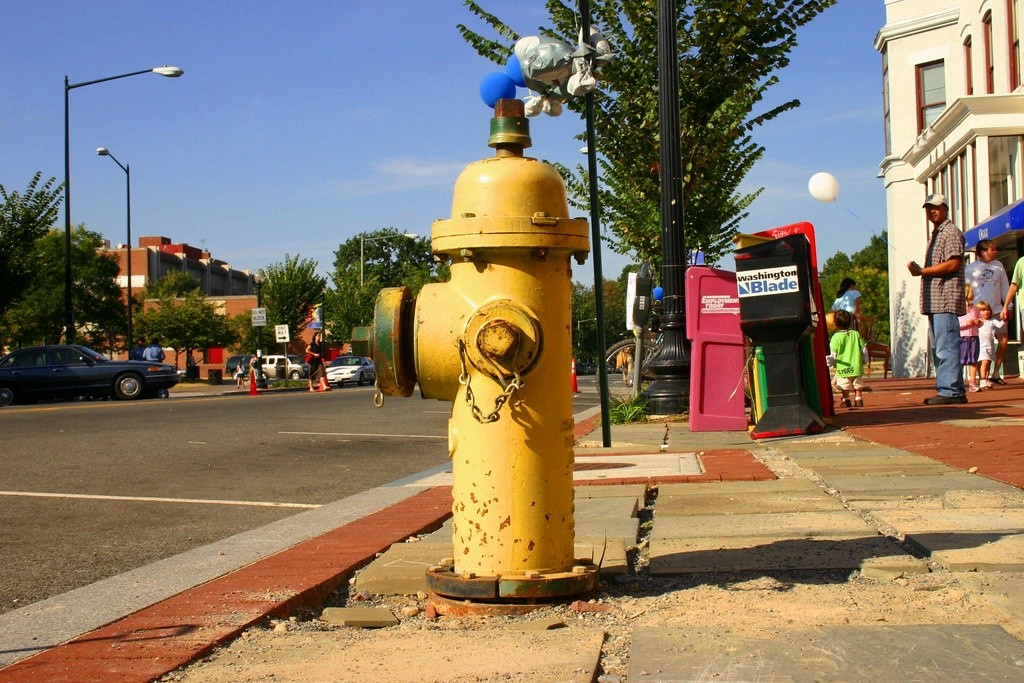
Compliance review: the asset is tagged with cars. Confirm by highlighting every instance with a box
[325,356,376,388]
[0,344,182,408]
[171,366,187,382]
[225,354,255,377]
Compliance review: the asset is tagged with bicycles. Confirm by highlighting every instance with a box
[596,332,665,411]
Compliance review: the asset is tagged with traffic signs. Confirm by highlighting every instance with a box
[275,324,290,343]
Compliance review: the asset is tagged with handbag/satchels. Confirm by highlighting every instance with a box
[305,353,312,361]
[234,373,238,380]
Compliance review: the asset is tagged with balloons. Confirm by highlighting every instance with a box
[651,287,665,304]
[479,53,537,112]
[808,171,841,202]
[824,312,840,332]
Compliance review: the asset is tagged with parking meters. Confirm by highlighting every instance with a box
[632,277,652,399]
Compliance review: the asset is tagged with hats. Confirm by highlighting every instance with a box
[922,194,949,210]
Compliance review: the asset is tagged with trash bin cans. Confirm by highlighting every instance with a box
[275,362,286,380]
[207,369,223,386]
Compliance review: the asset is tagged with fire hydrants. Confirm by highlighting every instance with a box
[351,97,601,618]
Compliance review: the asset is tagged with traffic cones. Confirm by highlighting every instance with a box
[571,357,582,394]
[247,370,261,396]
[317,358,331,391]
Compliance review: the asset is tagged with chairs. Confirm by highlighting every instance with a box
[52,351,65,365]
[860,315,890,379]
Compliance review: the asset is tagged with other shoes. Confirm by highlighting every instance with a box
[924,395,967,405]
[969,383,982,392]
[840,400,851,407]
[324,386,330,391]
[855,400,863,406]
[989,378,1007,385]
[980,380,993,388]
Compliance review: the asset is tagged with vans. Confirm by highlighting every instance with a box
[261,354,311,380]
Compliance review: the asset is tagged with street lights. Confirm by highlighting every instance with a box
[254,271,269,389]
[96,146,132,361]
[64,64,185,345]
[360,233,420,287]
[578,318,597,347]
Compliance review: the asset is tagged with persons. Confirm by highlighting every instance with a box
[955,284,984,392]
[906,193,967,405]
[825,309,869,409]
[305,332,331,393]
[143,338,170,399]
[188,351,197,384]
[249,353,260,388]
[2,346,11,358]
[236,359,245,389]
[964,239,1013,385]
[1000,255,1024,333]
[129,337,148,361]
[616,345,634,387]
[831,277,863,376]
[975,301,1007,388]
